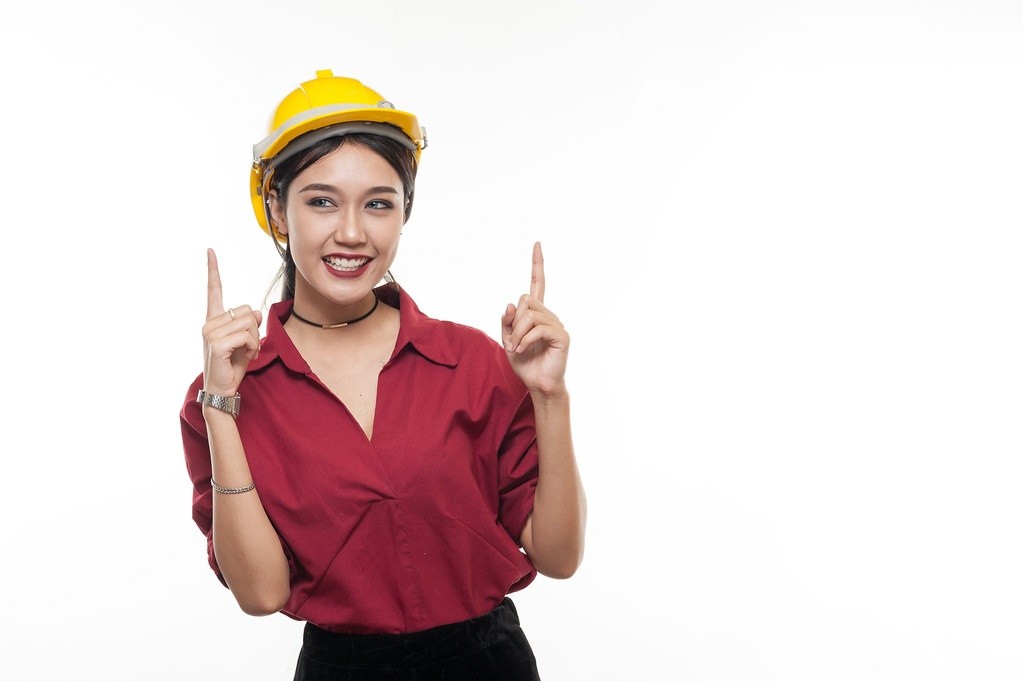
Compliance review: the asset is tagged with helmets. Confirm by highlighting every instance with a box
[251,71,428,245]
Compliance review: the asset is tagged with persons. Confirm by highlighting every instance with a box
[177,70,587,681]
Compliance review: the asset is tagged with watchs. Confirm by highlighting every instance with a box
[196,389,241,420]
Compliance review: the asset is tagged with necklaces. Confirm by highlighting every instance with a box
[290,295,379,330]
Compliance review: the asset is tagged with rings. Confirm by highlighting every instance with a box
[229,308,235,320]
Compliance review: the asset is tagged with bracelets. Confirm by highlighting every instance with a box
[210,478,255,495]
